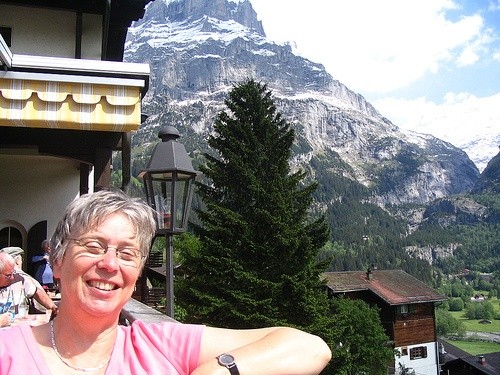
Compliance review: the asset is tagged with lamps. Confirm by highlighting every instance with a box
[145,125,197,319]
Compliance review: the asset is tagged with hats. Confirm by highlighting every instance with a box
[2,247,24,257]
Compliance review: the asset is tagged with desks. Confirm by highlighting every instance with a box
[1,314,50,327]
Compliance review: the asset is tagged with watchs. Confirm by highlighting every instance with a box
[215,353,239,375]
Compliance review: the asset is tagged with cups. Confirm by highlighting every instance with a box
[18,305,29,318]
[3,306,15,324]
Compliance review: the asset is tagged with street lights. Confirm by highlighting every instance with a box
[143,127,198,318]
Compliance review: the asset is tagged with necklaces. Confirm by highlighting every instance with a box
[49,318,111,372]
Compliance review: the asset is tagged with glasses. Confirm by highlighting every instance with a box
[0,271,16,279]
[66,237,146,267]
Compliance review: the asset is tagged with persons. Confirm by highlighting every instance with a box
[1,247,47,314]
[28,240,54,292]
[0,252,57,327]
[0,190,331,375]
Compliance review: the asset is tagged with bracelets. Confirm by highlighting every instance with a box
[53,306,58,312]
